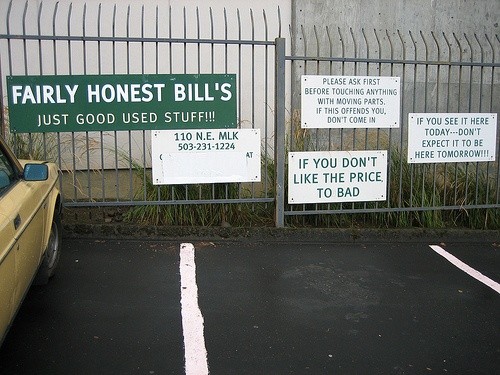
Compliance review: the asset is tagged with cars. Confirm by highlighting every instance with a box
[0,133,63,347]
